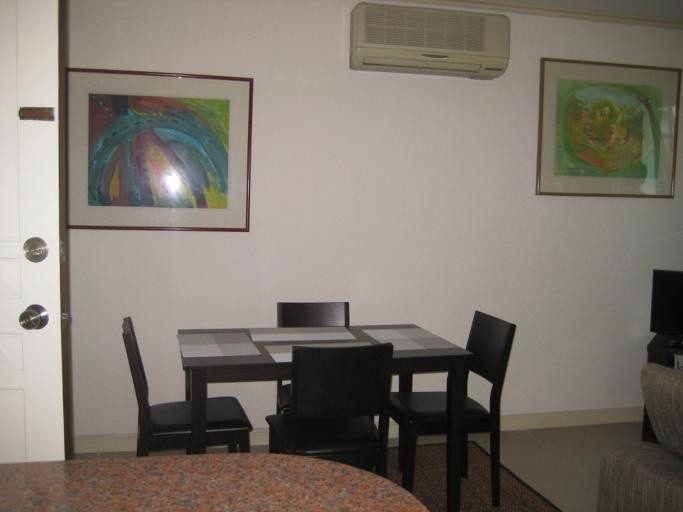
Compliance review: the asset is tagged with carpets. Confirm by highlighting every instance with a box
[206,440,563,512]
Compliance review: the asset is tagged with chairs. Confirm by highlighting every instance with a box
[390,310,516,507]
[276,302,350,412]
[122,317,252,453]
[265,342,392,477]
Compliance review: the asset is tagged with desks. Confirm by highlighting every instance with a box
[0,452,430,512]
[178,323,476,512]
[642,333,683,445]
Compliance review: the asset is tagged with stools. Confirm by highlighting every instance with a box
[593,445,683,512]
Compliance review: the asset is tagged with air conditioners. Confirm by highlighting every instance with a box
[349,4,513,80]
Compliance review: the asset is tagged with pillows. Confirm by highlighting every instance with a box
[640,362,683,457]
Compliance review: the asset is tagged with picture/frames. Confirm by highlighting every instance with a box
[64,67,253,232]
[537,57,681,196]
